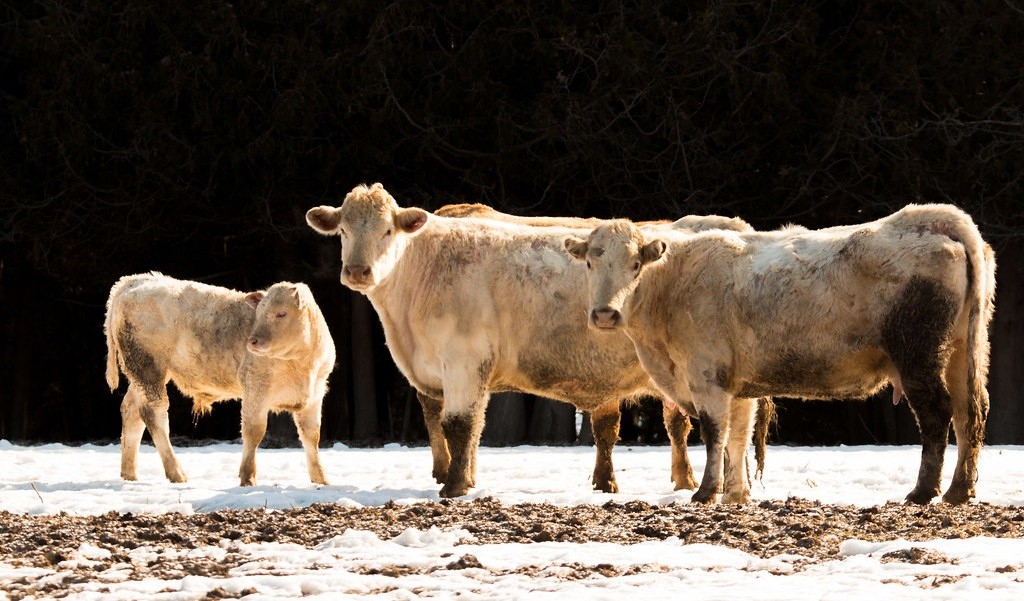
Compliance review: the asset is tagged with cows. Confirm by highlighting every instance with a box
[103,270,335,487]
[305,183,776,498]
[565,203,997,506]
